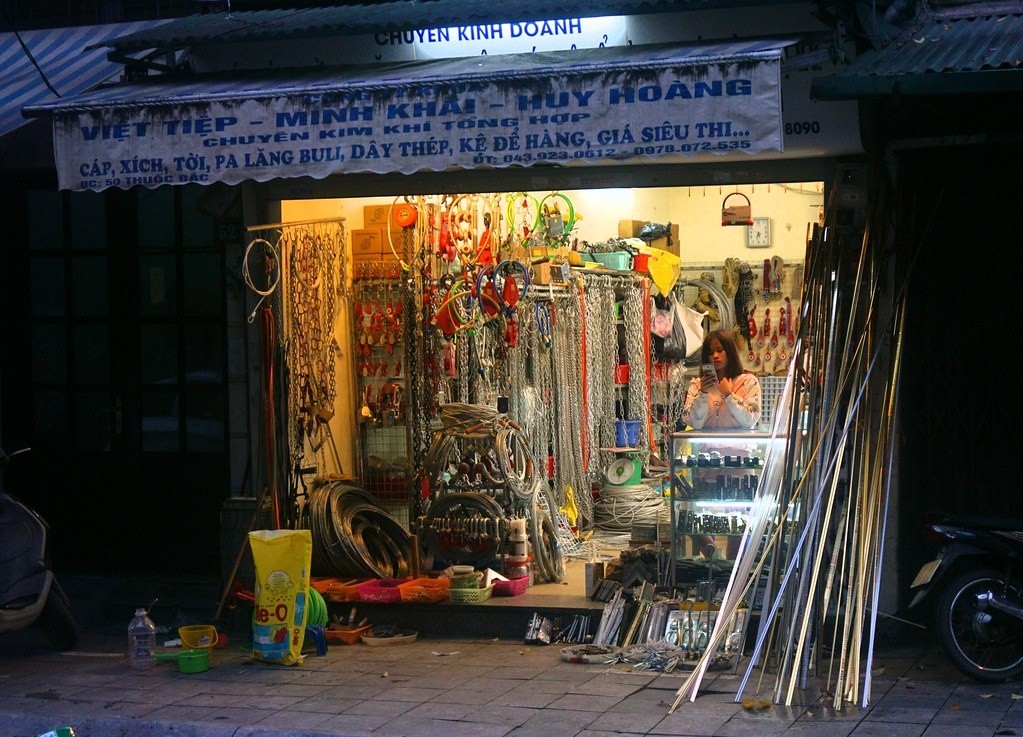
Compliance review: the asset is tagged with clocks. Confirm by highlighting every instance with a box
[747,217,771,248]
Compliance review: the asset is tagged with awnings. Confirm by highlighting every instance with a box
[810,16,1022,103]
[0,16,192,134]
[82,0,755,53]
[21,35,828,192]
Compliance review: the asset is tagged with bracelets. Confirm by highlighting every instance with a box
[699,389,708,394]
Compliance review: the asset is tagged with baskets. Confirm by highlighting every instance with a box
[357,578,412,603]
[634,253,652,273]
[323,623,374,644]
[307,574,334,594]
[368,472,429,500]
[317,576,377,601]
[398,576,453,602]
[653,362,671,382]
[616,420,642,448]
[491,575,531,595]
[446,580,496,604]
[580,251,632,272]
[614,365,630,384]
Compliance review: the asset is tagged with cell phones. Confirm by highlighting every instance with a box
[702,363,718,382]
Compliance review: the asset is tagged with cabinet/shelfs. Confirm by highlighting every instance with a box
[353,268,670,535]
[670,429,808,586]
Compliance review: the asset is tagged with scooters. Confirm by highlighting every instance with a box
[0,447,83,653]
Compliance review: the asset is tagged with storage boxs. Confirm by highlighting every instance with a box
[352,203,441,281]
[500,241,568,285]
[618,220,680,257]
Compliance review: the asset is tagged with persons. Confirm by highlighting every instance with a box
[682,329,762,561]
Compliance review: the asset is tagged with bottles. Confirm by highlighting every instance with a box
[128,607,156,671]
[37,726,77,737]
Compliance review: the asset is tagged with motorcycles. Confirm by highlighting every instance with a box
[905,511,1023,685]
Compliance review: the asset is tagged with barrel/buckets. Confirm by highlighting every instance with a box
[178,624,218,659]
